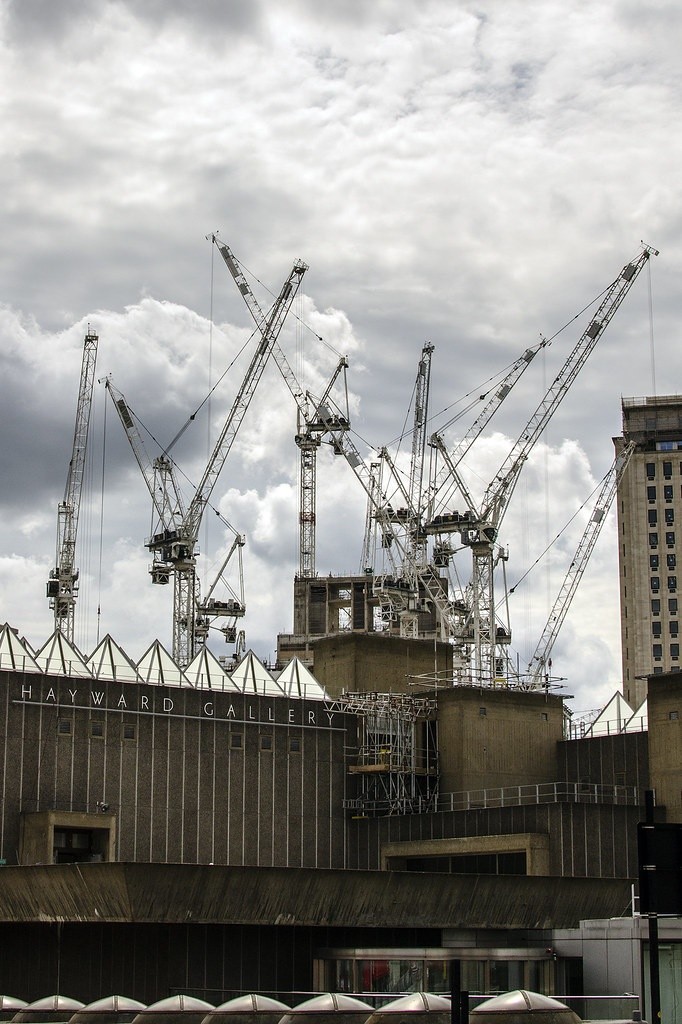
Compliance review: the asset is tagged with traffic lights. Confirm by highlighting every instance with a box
[638,821,682,913]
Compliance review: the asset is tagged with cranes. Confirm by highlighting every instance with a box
[46,324,98,643]
[101,234,659,694]
